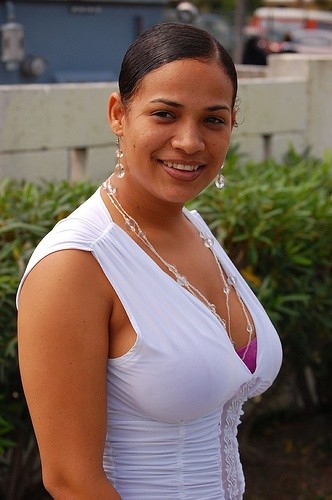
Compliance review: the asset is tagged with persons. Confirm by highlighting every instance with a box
[15,22,282,500]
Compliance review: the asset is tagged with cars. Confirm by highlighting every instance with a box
[236,8,332,65]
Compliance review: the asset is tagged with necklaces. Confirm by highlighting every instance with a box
[101,172,252,363]
